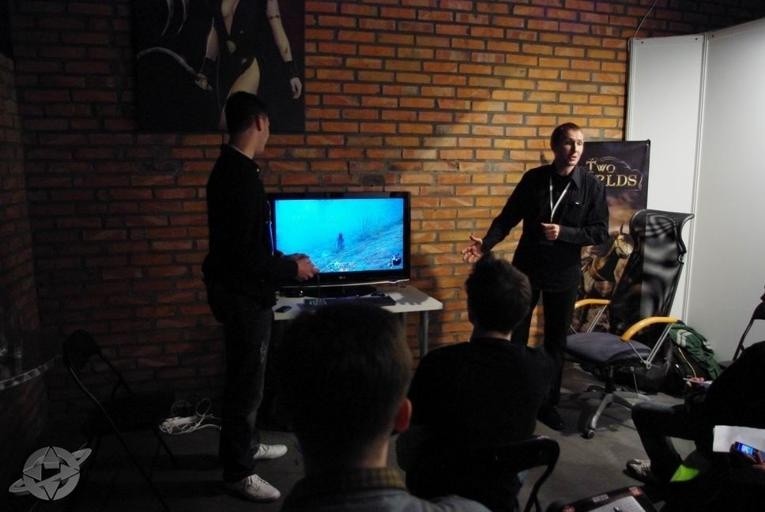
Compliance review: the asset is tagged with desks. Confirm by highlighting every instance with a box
[268,285,444,429]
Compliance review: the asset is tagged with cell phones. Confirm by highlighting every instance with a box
[733,441,762,464]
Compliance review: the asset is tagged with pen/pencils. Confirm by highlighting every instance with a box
[612,508,621,512]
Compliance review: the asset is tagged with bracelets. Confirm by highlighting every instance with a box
[282,59,304,85]
[199,56,214,76]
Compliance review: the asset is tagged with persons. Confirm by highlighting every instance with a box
[200,89,318,505]
[458,119,614,436]
[272,300,495,512]
[658,437,765,512]
[621,337,765,483]
[392,257,566,512]
[193,0,304,132]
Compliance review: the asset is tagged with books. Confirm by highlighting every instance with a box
[711,423,765,456]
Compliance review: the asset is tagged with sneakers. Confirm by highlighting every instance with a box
[626,458,651,481]
[252,442,288,460]
[539,408,567,430]
[232,473,282,501]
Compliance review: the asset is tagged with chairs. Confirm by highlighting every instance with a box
[565,208,695,439]
[27,332,177,511]
[717,293,765,371]
[461,435,560,510]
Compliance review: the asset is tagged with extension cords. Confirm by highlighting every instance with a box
[161,415,198,429]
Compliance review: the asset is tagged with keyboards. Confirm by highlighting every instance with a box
[303,294,396,311]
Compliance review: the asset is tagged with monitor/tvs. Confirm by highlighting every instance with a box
[267,191,411,299]
